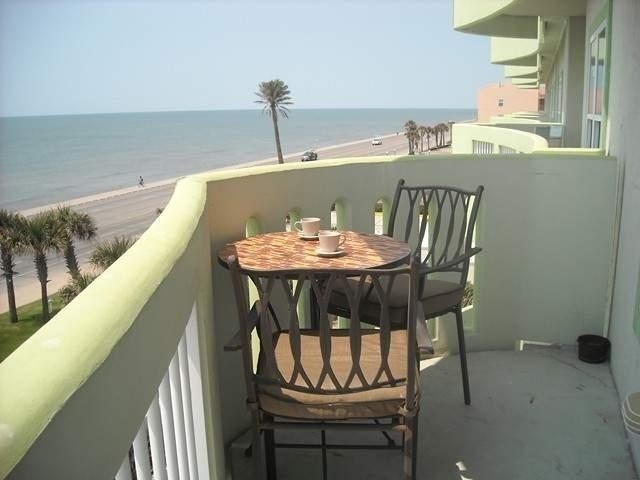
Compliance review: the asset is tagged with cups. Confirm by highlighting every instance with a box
[318,230,347,251]
[294,217,320,236]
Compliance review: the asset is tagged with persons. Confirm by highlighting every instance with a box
[139,175,144,187]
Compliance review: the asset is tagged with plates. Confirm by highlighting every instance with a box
[315,247,345,257]
[296,233,319,240]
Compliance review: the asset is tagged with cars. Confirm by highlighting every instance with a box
[301,151,318,162]
[372,138,383,145]
